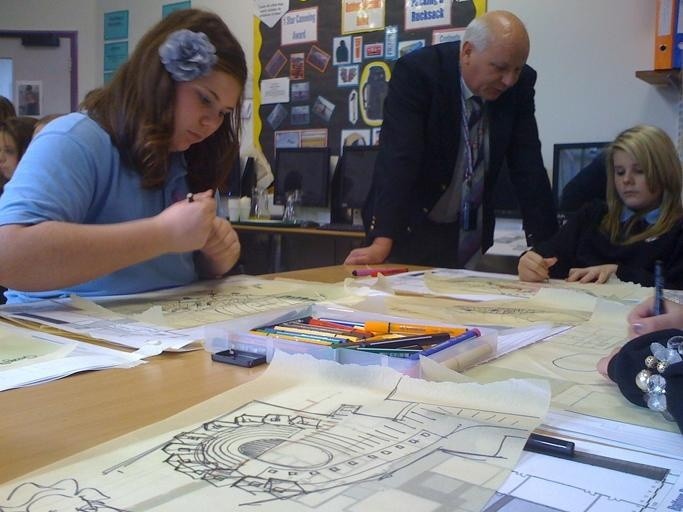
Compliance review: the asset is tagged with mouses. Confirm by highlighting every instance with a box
[301,221,319,228]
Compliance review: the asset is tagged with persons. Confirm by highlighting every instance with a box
[517,124,683,291]
[0,85,101,195]
[0,7,249,304]
[595,294,683,437]
[343,9,562,271]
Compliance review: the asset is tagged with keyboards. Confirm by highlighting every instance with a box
[317,223,366,232]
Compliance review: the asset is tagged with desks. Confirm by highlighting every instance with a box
[226,224,366,272]
[0,264,683,511]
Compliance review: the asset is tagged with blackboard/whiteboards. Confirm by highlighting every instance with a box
[252,1,487,195]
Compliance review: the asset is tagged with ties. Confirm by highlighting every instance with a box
[459,101,484,268]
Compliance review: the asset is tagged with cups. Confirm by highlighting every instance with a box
[240,198,251,222]
[256,188,271,219]
[228,199,239,222]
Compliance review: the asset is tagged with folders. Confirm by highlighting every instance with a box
[653,0,682,70]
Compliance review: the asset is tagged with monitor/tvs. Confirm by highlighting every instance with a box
[339,145,379,209]
[274,147,331,208]
[220,153,240,197]
[552,142,614,215]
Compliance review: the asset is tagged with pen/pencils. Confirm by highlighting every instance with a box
[250,316,492,361]
[524,432,575,458]
[527,233,551,283]
[352,267,408,277]
[655,260,663,317]
[186,193,193,203]
